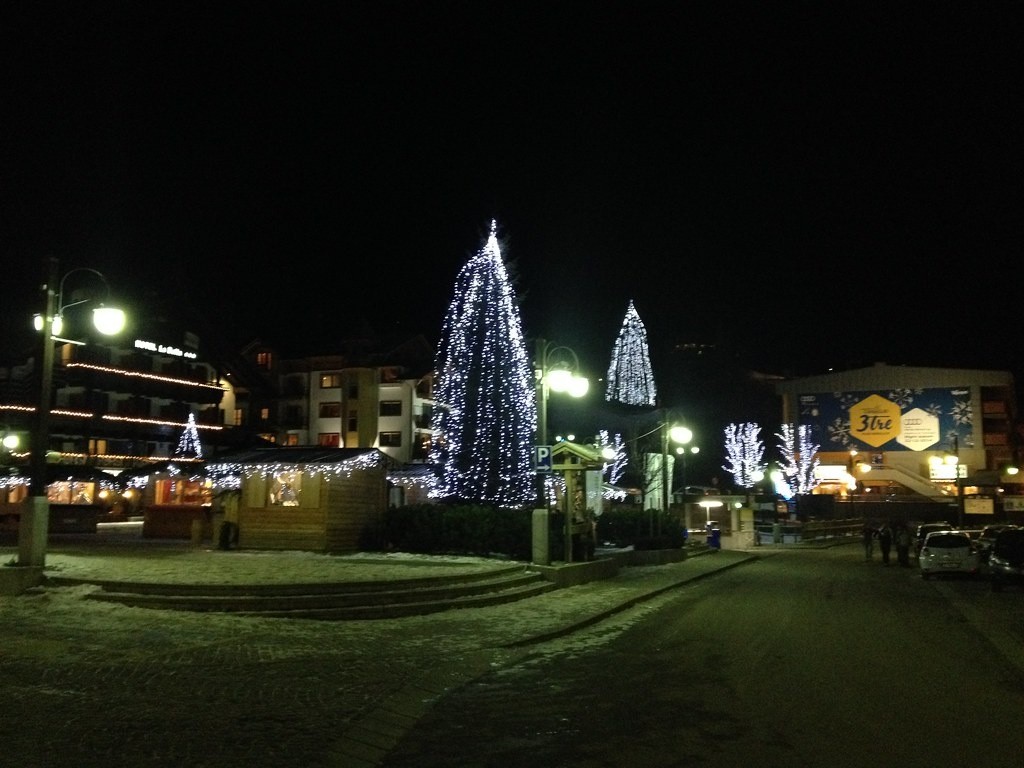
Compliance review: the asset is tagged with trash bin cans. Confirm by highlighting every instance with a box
[706,521,721,550]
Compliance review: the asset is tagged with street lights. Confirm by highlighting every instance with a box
[18,264,128,567]
[659,407,701,517]
[534,340,590,507]
[848,450,872,519]
[929,443,964,531]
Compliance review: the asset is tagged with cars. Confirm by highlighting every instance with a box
[916,530,977,581]
[910,523,946,551]
[980,524,1018,551]
[988,529,1024,593]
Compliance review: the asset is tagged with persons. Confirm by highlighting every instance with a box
[861,519,911,568]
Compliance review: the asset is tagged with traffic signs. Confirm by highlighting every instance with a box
[534,446,553,474]
